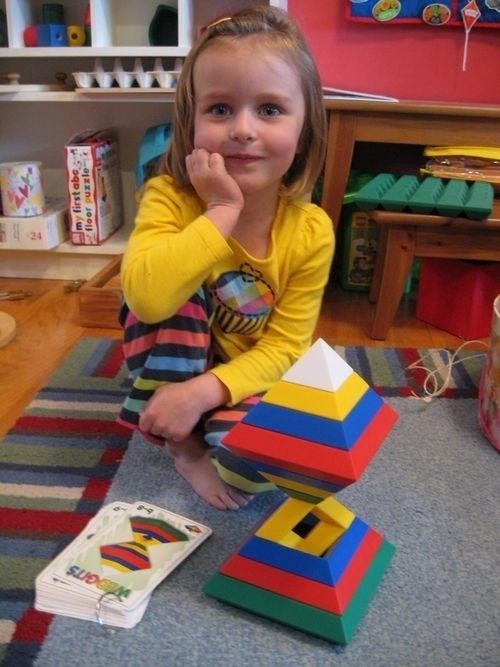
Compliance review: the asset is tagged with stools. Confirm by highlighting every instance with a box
[367,199,499,340]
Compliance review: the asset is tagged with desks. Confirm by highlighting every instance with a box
[310,95,500,233]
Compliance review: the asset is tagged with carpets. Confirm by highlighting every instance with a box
[1,337,496,665]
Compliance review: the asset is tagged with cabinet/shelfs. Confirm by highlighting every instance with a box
[1,0,288,278]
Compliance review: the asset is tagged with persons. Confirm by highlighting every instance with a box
[115,3,335,510]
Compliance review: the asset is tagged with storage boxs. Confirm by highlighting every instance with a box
[415,257,500,339]
[0,195,68,251]
[62,127,126,247]
